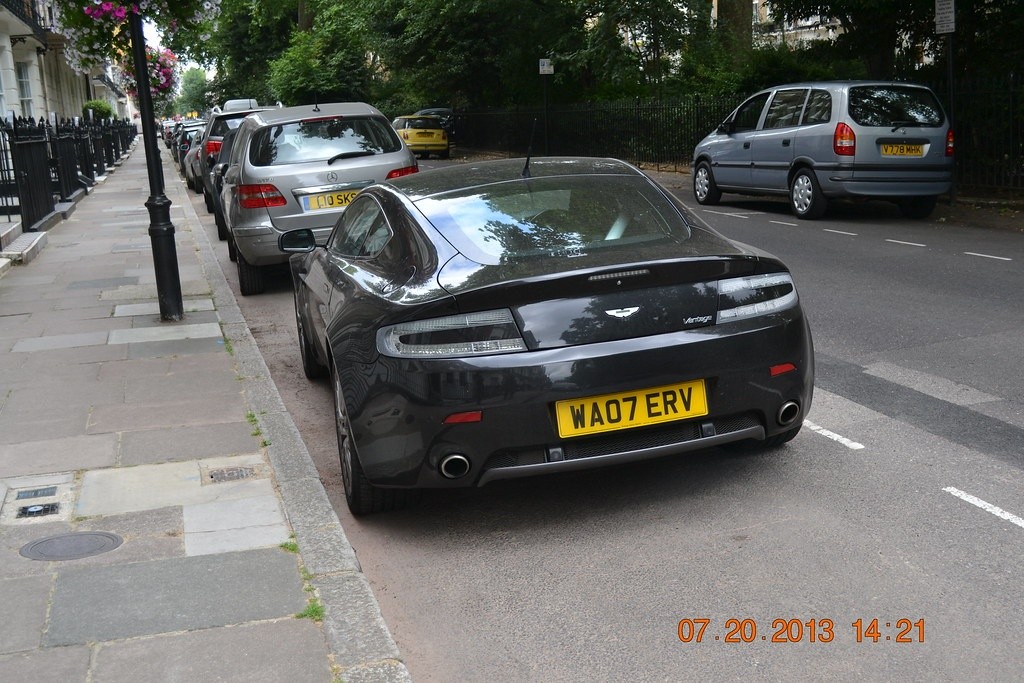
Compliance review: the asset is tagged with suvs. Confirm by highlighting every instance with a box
[200,99,288,213]
[392,114,450,157]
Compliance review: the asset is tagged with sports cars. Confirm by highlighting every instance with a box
[276,155,815,518]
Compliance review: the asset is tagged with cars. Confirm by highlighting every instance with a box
[411,108,457,142]
[208,128,237,241]
[211,101,419,294]
[689,79,955,219]
[156,118,207,194]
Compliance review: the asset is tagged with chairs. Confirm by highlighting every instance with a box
[564,184,647,245]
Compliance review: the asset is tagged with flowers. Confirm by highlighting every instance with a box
[120,44,179,110]
[49,0,221,77]
